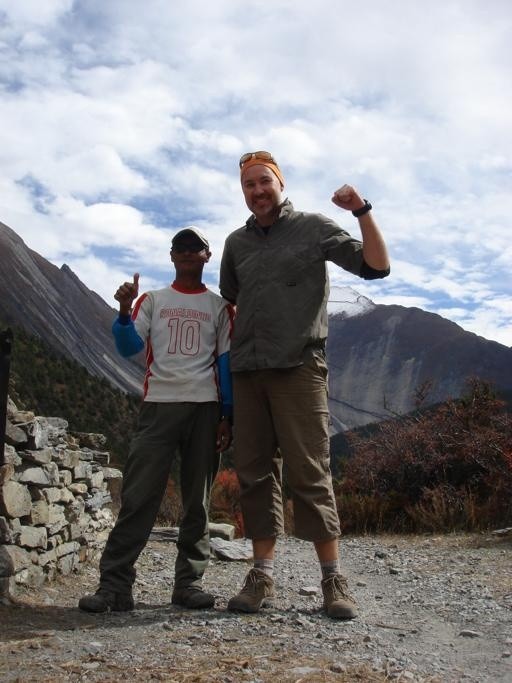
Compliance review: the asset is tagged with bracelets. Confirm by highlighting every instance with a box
[351,198,372,217]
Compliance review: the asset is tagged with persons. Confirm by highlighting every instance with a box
[78,225,233,613]
[219,150,390,620]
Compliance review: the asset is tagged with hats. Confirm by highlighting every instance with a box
[172,226,209,248]
[240,154,284,186]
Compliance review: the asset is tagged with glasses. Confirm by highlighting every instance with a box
[240,150,277,168]
[171,242,204,252]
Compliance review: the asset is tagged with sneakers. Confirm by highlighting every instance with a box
[172,587,215,608]
[79,588,134,612]
[320,572,356,619]
[228,570,273,612]
